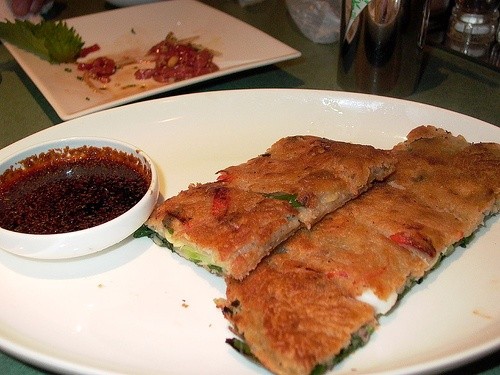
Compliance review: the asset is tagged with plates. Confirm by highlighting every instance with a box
[0,0,304,122]
[0,87,499,375]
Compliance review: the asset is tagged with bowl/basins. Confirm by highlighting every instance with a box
[0,136,160,260]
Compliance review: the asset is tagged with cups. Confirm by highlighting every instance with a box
[448,5,497,61]
[285,1,341,44]
[336,0,431,99]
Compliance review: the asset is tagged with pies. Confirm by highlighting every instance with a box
[145,125,499,373]
